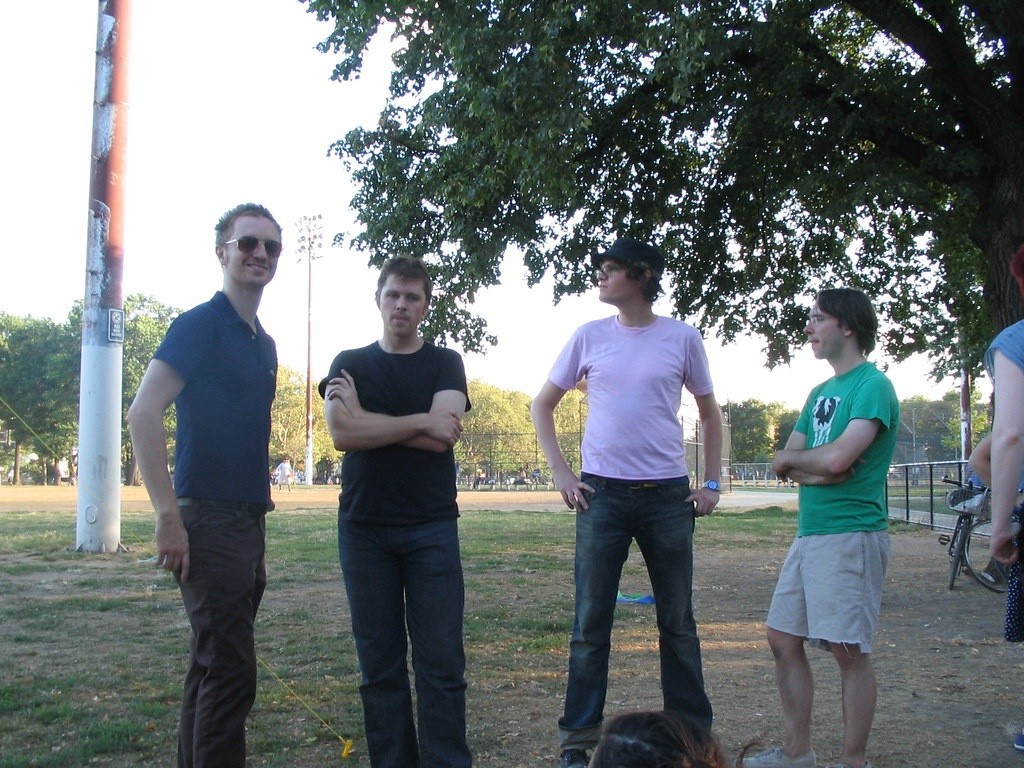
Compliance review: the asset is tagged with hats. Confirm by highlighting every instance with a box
[591,237,664,278]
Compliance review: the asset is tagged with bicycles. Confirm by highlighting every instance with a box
[963,517,1018,594]
[938,475,994,591]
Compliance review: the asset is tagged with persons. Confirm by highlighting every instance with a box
[586,710,726,768]
[318,254,473,768]
[126,201,282,768]
[735,289,900,768]
[529,236,723,768]
[459,467,541,490]
[967,250,1024,751]
[267,456,305,493]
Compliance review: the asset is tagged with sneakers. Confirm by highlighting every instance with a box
[823,760,871,768]
[734,748,817,768]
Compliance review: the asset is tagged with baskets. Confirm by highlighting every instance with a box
[946,486,986,514]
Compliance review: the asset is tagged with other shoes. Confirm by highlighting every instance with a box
[560,749,590,768]
[1014,734,1024,750]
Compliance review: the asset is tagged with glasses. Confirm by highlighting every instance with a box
[805,316,835,327]
[594,268,612,278]
[226,235,282,258]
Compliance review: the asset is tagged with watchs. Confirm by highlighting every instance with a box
[702,479,721,494]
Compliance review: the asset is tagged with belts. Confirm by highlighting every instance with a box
[177,496,266,514]
[590,476,659,491]
